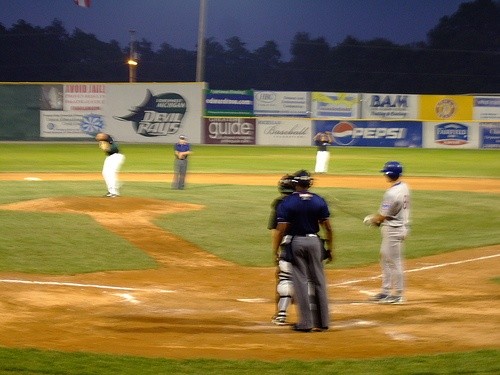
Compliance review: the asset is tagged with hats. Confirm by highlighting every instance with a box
[179,135,184,140]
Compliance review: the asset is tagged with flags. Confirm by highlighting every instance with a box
[73,0,91,8]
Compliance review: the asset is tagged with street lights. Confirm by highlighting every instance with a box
[127,29,138,82]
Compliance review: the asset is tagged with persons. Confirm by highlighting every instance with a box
[364,161,409,304]
[268,170,333,333]
[314,131,331,173]
[173,136,191,190]
[96,133,123,197]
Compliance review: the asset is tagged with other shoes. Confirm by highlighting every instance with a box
[370,291,404,304]
[106,192,120,199]
[291,324,329,333]
[272,318,287,327]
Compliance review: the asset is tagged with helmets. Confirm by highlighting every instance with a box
[292,170,313,186]
[278,175,294,189]
[380,161,403,179]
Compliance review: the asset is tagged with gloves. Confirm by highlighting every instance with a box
[363,214,375,226]
